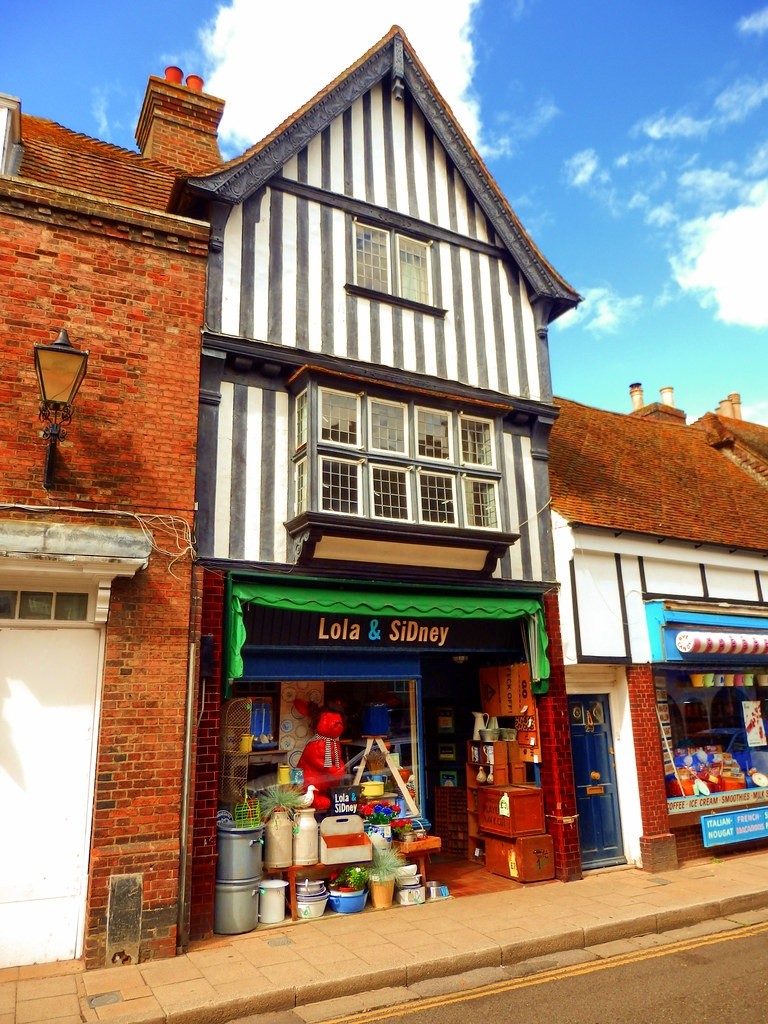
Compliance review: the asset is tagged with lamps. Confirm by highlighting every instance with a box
[33,329,89,489]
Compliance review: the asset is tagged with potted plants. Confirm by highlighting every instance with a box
[258,783,300,821]
[332,865,369,892]
[368,847,407,909]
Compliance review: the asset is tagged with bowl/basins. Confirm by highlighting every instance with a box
[360,782,384,796]
[478,728,502,741]
[294,877,330,919]
[328,888,368,912]
[426,881,447,898]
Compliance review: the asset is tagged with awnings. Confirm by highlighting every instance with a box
[675,630,768,655]
[228,584,551,697]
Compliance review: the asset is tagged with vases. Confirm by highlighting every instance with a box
[400,832,420,842]
[366,824,392,849]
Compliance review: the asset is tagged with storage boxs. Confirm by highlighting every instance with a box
[486,833,555,882]
[477,785,545,838]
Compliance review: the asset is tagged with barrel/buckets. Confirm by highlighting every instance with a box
[213,830,290,935]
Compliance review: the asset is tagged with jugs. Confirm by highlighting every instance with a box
[293,808,319,866]
[471,743,494,765]
[366,775,388,784]
[471,711,489,740]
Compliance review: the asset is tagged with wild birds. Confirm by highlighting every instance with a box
[296,785,319,809]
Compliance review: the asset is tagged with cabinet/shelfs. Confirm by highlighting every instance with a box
[434,742,526,866]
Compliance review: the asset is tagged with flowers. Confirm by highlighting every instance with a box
[360,800,401,825]
[391,818,415,833]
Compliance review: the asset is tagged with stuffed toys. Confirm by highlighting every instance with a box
[296,711,345,810]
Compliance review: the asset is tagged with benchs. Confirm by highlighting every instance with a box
[262,836,442,918]
